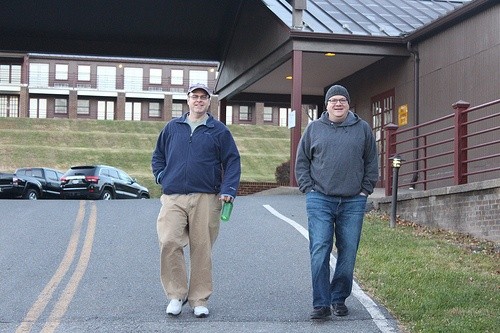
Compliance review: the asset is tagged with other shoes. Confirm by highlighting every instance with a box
[332,303,348,316]
[310,305,331,319]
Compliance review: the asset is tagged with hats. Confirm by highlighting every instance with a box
[325,85,350,106]
[186,83,210,95]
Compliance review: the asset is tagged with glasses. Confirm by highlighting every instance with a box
[327,99,347,104]
[190,94,209,100]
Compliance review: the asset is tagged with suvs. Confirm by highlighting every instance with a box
[60,165,150,200]
[15,167,64,198]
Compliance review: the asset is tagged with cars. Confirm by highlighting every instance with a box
[0,173,43,200]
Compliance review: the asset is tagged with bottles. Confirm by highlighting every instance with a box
[221,200,233,221]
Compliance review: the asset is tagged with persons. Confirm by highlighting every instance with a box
[295,85,379,319]
[151,82,241,318]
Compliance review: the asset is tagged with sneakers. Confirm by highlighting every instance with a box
[193,306,210,318]
[166,296,188,316]
